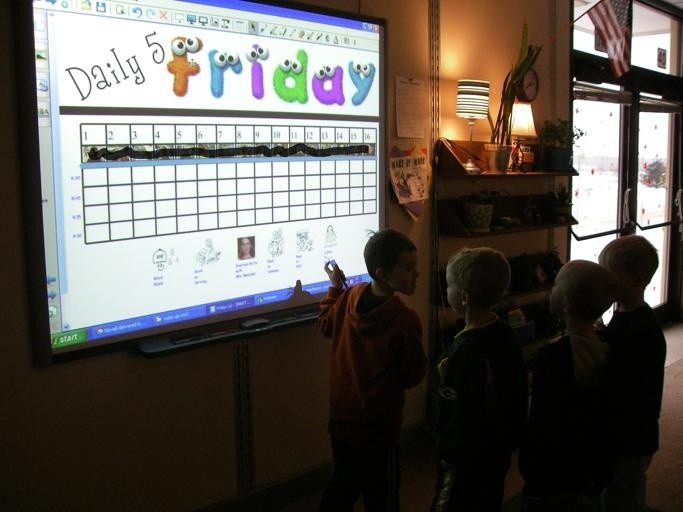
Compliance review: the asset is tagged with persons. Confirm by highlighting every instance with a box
[432,244,528,512]
[524,257,636,511]
[237,237,253,260]
[317,226,426,512]
[598,234,666,510]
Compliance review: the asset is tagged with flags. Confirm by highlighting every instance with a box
[587,1,633,78]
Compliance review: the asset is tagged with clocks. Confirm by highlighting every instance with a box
[515,67,540,103]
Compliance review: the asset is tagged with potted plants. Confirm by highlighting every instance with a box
[484,19,545,175]
[539,119,584,172]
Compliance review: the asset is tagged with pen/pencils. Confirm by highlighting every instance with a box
[330,260,348,288]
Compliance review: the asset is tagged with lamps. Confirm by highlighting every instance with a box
[457,78,491,173]
[506,102,538,173]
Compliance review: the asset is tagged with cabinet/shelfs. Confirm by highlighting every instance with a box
[426,132,585,399]
